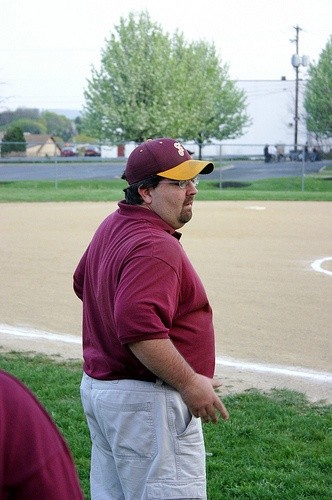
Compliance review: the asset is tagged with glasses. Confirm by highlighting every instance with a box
[148,177,199,189]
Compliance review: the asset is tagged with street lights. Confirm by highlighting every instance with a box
[288,25,311,152]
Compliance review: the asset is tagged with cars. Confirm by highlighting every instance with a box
[83,145,102,157]
[60,146,78,157]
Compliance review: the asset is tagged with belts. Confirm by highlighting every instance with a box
[126,374,170,387]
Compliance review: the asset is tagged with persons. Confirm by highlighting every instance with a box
[0,369,86,500]
[73,139,230,500]
[275,145,281,162]
[303,142,322,162]
[264,144,271,163]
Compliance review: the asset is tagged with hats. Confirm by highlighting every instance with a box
[125,138,215,185]
